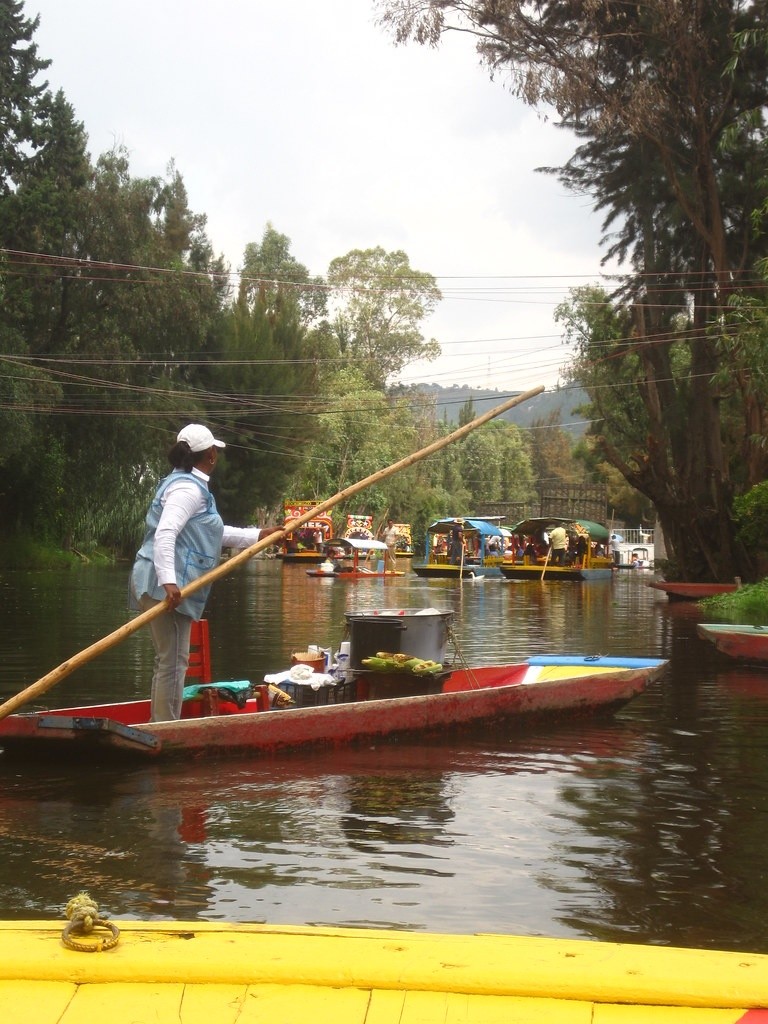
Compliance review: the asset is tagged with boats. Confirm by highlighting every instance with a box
[696,622,768,663]
[0,657,676,762]
[273,501,336,562]
[645,580,751,598]
[390,523,414,558]
[631,547,653,569]
[331,514,381,559]
[304,538,406,580]
[410,516,511,577]
[501,516,619,582]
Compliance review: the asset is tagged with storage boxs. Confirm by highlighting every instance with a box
[264,679,359,710]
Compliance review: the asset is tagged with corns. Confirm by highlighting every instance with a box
[362,651,443,675]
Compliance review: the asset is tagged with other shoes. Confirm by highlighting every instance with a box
[463,564,467,566]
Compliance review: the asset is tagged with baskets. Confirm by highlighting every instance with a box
[278,678,357,706]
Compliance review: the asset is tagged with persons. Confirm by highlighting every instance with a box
[608,535,620,564]
[129,423,289,725]
[449,518,466,566]
[312,527,323,553]
[549,522,567,567]
[379,518,400,573]
[324,549,352,572]
[569,536,586,560]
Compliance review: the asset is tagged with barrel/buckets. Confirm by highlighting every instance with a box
[350,617,407,671]
[344,608,455,666]
[291,652,326,674]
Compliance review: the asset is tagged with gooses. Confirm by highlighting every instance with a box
[467,571,485,580]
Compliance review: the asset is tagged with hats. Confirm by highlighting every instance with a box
[177,423,226,453]
[454,518,465,523]
[315,528,320,530]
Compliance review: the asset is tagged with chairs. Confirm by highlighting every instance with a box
[180,619,269,720]
